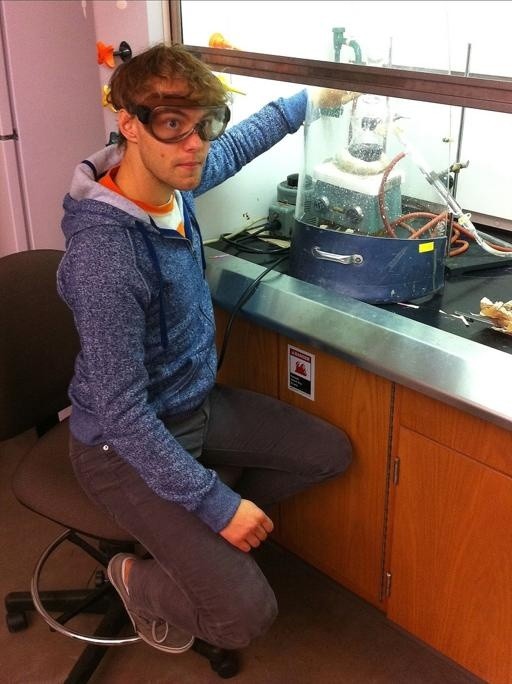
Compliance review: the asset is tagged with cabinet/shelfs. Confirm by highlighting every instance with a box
[387,380,512,684]
[209,299,402,630]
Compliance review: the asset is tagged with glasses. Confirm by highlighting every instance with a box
[125,99,232,143]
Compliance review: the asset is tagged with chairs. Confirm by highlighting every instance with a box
[1,249,271,680]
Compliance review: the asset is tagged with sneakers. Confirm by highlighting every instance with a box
[106,552,195,655]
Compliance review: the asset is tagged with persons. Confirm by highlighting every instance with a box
[52,42,363,655]
